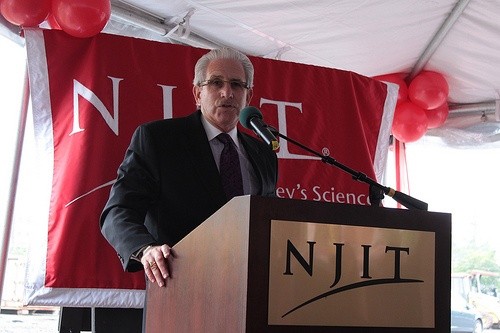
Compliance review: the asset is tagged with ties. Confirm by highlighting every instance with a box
[215,133,243,201]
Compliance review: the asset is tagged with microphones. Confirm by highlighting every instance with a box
[239,106,278,147]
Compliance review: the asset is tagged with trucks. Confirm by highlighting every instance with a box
[451,269,499,329]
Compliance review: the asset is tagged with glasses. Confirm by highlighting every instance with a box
[201,79,250,89]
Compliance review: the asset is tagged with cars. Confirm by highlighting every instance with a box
[450,284,484,333]
